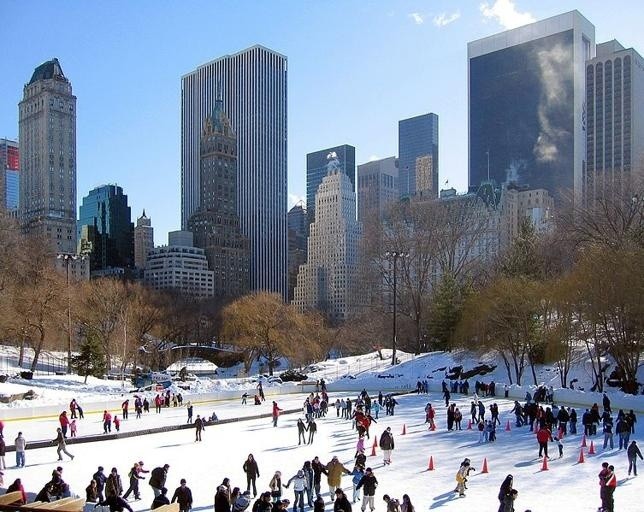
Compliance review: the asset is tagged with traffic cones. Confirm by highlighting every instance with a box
[539,455,550,471]
[587,441,596,455]
[533,422,539,434]
[369,445,377,457]
[579,434,588,448]
[576,448,586,464]
[427,456,436,471]
[466,419,472,430]
[401,423,407,436]
[505,419,512,431]
[481,458,490,474]
[373,435,377,448]
[557,426,564,439]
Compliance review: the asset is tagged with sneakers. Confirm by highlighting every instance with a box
[15,465,24,467]
[598,507,607,512]
[459,493,466,496]
[546,456,550,459]
[353,498,360,503]
[384,460,391,465]
[539,455,543,458]
[71,456,74,461]
[135,497,141,500]
[454,490,460,492]
[57,458,63,461]
[628,472,638,476]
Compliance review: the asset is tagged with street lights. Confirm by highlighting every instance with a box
[57,252,86,375]
[383,250,410,365]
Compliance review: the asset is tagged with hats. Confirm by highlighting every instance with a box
[609,464,614,472]
[601,462,609,468]
[98,466,104,471]
[138,461,144,465]
[180,478,186,484]
[297,469,305,476]
[111,467,117,472]
[219,484,228,493]
[134,463,139,467]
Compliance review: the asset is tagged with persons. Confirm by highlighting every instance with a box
[2,374,643,510]
[177,365,188,382]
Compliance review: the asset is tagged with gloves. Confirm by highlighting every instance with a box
[146,471,149,473]
[472,467,475,471]
[142,477,145,479]
[463,478,468,482]
